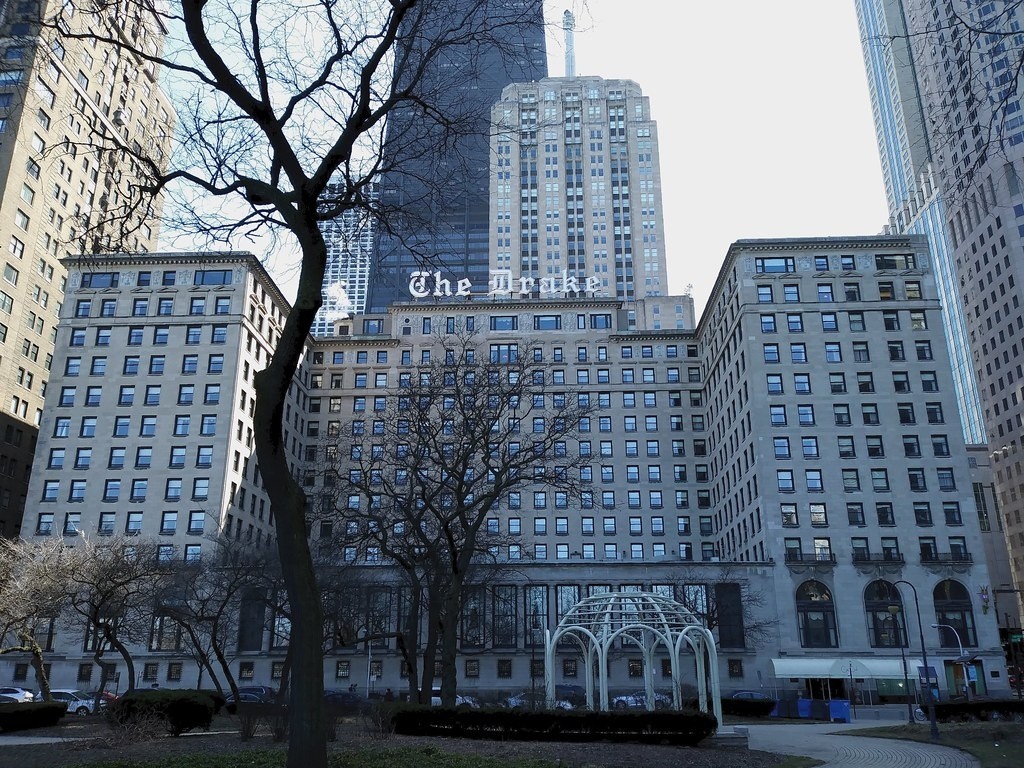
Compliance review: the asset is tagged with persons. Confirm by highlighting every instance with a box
[348,684,358,692]
[384,688,393,703]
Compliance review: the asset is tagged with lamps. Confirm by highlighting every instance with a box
[977,584,992,614]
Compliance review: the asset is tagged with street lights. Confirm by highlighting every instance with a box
[881,616,916,725]
[888,581,941,739]
[848,661,858,719]
[361,628,372,698]
[931,624,972,703]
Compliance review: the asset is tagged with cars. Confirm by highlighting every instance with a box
[0,685,480,716]
[610,691,672,710]
[507,692,572,711]
[721,689,771,701]
